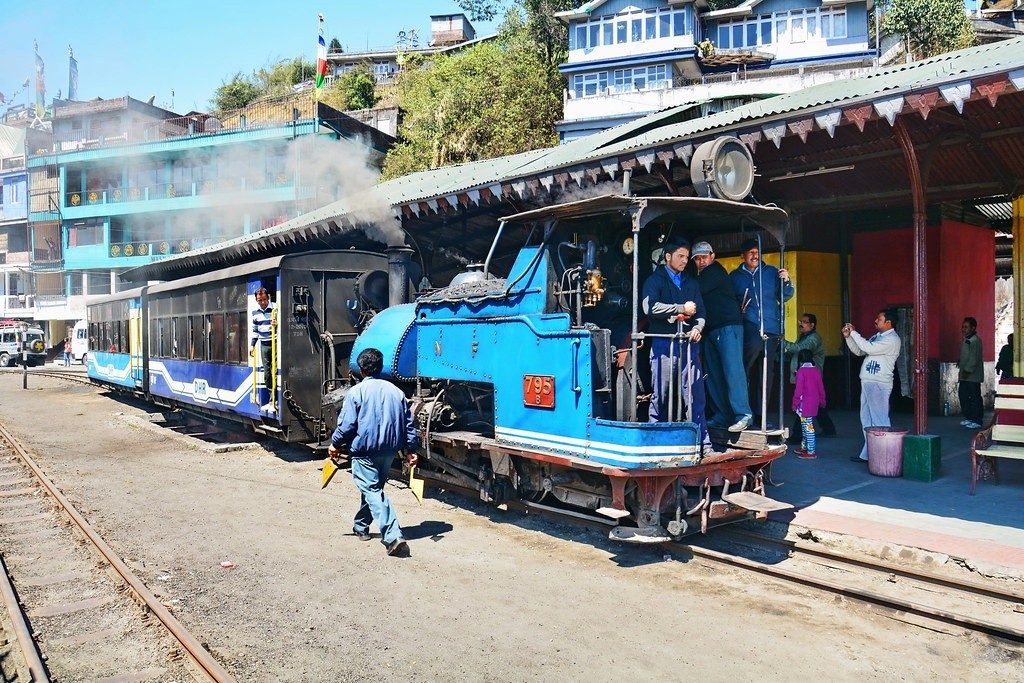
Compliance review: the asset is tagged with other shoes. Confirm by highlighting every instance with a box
[261,402,272,411]
[849,456,869,462]
[353,527,370,540]
[798,452,817,458]
[794,448,808,454]
[269,404,278,412]
[388,536,406,554]
[706,418,729,429]
[965,422,981,428]
[727,413,753,431]
[960,420,973,425]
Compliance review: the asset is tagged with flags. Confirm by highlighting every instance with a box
[318,32,328,90]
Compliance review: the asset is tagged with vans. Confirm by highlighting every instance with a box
[71,319,87,365]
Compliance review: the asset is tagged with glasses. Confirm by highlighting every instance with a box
[798,320,813,324]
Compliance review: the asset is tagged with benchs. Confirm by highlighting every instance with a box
[970,384,1024,495]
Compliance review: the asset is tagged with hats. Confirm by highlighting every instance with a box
[740,238,760,251]
[691,241,714,258]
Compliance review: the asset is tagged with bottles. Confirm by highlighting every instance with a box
[944,400,949,417]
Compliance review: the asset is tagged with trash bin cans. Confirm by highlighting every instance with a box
[864,425,910,478]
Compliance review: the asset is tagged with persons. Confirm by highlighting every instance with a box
[64,338,71,367]
[642,238,836,459]
[842,311,902,462]
[90,336,95,343]
[994,334,1015,380]
[249,287,279,401]
[328,348,421,554]
[956,317,985,428]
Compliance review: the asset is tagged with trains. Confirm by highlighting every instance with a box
[85,132,798,545]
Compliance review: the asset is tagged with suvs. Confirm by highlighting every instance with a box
[0,320,48,367]
